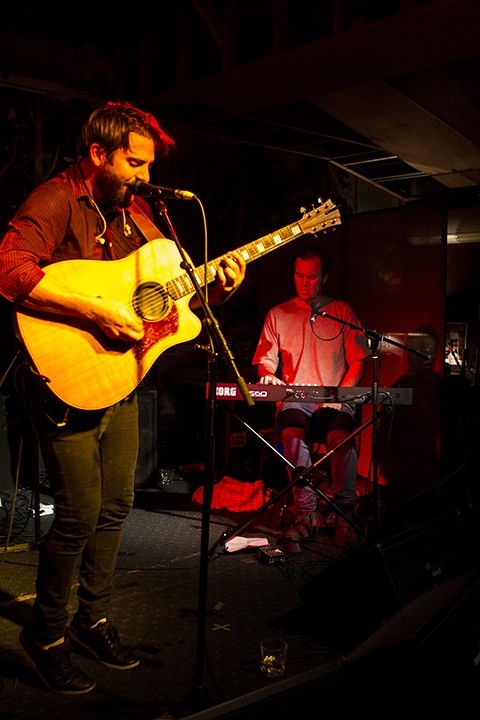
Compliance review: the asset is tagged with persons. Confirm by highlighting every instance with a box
[0,103,245,693]
[251,244,374,548]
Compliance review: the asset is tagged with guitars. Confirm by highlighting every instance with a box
[13,194,346,420]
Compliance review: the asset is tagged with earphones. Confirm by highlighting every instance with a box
[95,233,105,244]
[124,225,131,236]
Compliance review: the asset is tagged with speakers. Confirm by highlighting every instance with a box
[134,388,158,489]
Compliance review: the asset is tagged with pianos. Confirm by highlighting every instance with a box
[202,378,415,566]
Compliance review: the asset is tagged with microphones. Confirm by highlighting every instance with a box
[310,300,321,323]
[128,180,197,201]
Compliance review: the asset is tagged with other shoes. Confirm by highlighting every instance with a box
[287,517,320,541]
[335,516,356,545]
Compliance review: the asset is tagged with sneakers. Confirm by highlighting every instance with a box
[16,629,96,695]
[68,613,139,669]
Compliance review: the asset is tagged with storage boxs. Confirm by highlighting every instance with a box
[222,366,279,467]
